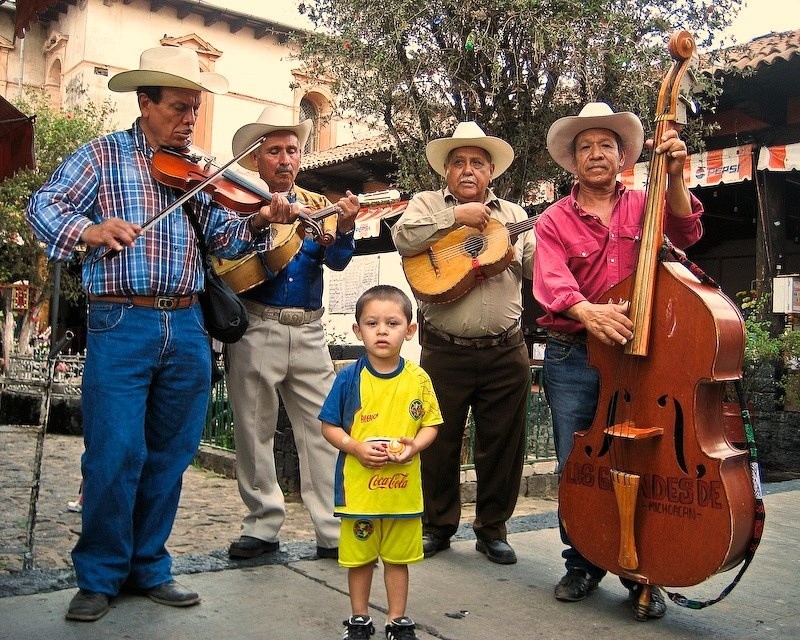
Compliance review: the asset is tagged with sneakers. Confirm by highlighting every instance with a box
[384,616,419,640]
[342,614,376,640]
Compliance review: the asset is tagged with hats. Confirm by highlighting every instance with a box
[425,121,514,180]
[108,46,231,95]
[231,107,314,172]
[546,102,644,175]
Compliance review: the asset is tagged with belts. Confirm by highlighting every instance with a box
[90,295,198,311]
[425,318,523,351]
[243,298,325,327]
[548,329,585,346]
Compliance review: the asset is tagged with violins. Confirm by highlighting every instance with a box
[149,143,337,247]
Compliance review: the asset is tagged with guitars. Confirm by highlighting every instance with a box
[208,188,403,296]
[400,213,542,306]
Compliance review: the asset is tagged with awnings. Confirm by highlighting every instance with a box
[347,143,800,235]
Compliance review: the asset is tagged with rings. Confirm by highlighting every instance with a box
[340,209,344,216]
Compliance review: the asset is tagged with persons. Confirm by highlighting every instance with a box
[22,47,360,621]
[317,285,442,640]
[533,102,705,619]
[391,123,536,562]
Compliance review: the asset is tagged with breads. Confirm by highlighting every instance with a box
[389,439,405,454]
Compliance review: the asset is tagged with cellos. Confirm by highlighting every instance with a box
[556,30,766,623]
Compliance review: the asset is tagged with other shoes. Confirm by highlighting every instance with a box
[317,547,379,564]
[421,532,450,557]
[629,584,667,621]
[476,537,517,565]
[66,589,110,621]
[229,535,280,558]
[137,579,201,606]
[554,567,598,601]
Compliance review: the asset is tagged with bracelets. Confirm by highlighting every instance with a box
[344,228,355,234]
